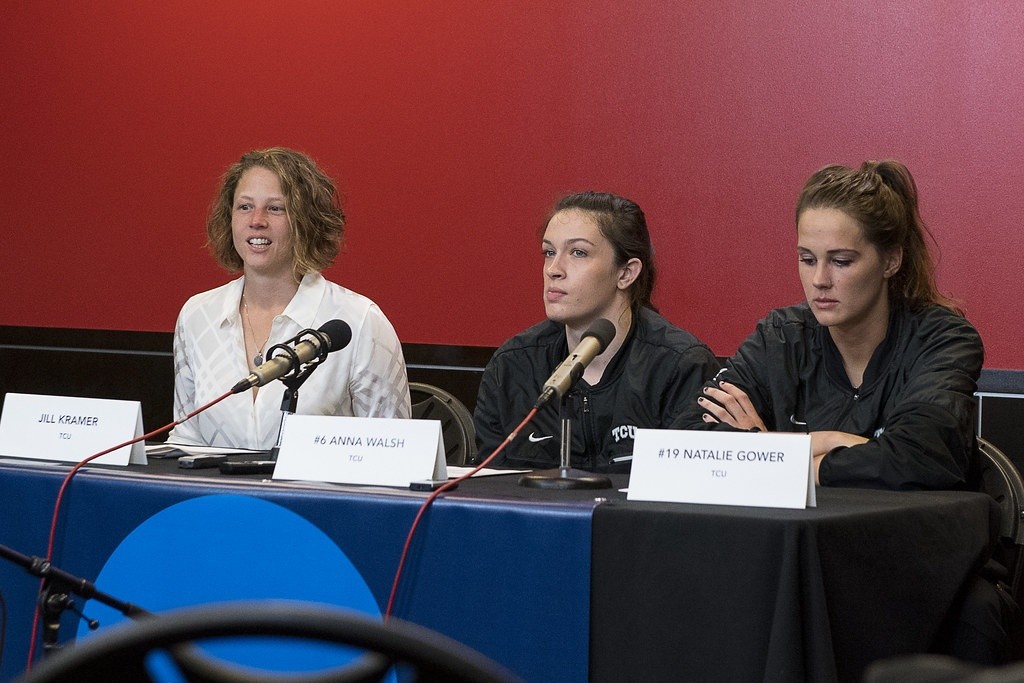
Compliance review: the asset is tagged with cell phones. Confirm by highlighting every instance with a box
[178,455,228,468]
[408,480,460,492]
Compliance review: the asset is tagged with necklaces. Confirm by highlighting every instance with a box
[242,294,269,367]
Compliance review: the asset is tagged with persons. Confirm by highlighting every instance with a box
[669,159,1024,667]
[165,146,413,451]
[471,189,721,472]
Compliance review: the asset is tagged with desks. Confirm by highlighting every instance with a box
[0,447,1001,683]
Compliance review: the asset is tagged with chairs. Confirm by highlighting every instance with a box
[974,435,1023,550]
[407,380,480,469]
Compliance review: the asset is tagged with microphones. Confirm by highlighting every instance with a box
[543,318,616,401]
[233,319,352,394]
